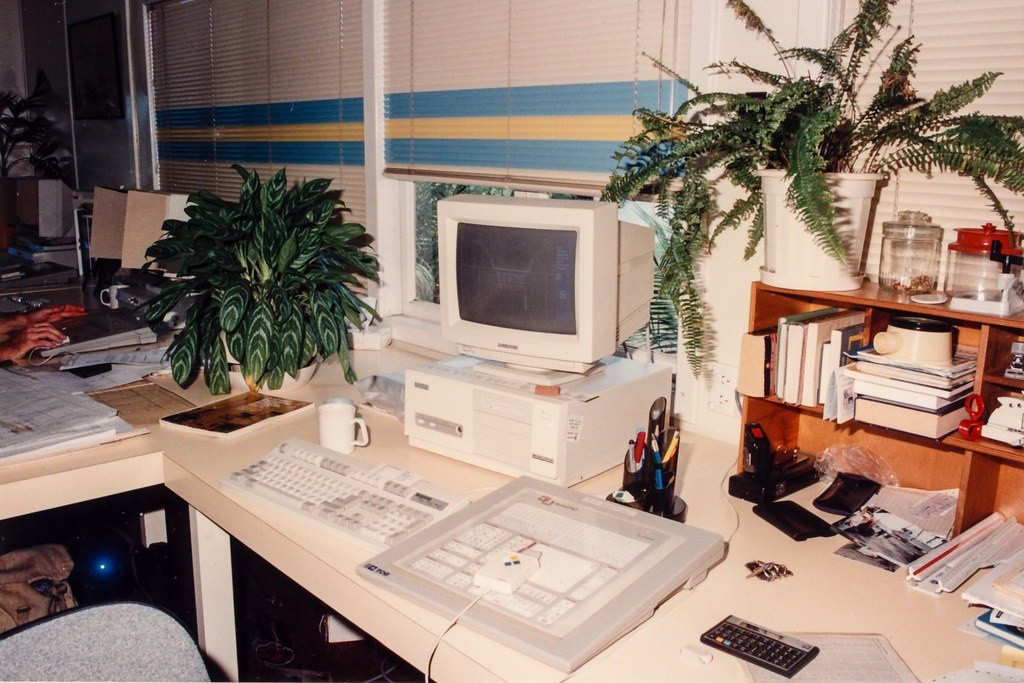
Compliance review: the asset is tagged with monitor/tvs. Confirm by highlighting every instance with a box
[36,177,94,246]
[437,194,656,387]
[121,189,202,281]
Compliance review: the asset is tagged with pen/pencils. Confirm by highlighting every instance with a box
[69,382,155,395]
[628,424,681,491]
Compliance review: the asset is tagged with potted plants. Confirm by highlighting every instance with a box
[132,161,382,396]
[602,1,1024,376]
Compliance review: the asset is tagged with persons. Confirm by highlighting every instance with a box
[0,304,89,362]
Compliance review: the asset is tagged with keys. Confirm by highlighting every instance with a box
[745,560,793,581]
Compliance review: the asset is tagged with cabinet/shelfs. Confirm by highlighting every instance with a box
[739,280,1024,540]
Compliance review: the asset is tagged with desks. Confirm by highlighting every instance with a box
[0,274,351,683]
[163,358,1024,683]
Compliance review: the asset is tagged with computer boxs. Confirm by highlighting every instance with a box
[404,356,672,489]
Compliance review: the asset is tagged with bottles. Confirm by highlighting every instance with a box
[879,209,944,296]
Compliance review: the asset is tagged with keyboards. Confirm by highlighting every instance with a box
[220,436,471,554]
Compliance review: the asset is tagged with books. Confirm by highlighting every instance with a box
[960,546,1024,650]
[40,310,157,358]
[843,344,975,441]
[159,390,315,440]
[736,306,864,407]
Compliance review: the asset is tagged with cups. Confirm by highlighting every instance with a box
[100,285,129,309]
[318,403,369,454]
